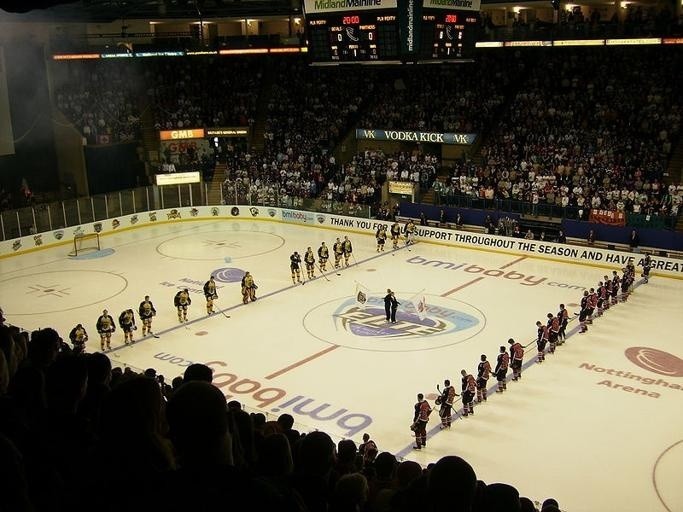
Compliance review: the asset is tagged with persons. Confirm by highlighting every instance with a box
[575,251,651,334]
[240,271,257,304]
[289,251,302,285]
[373,224,385,253]
[340,233,353,267]
[389,293,400,324]
[45,9,682,248]
[173,289,191,323]
[0,308,563,511]
[303,246,316,280]
[557,304,571,342]
[381,288,391,323]
[316,242,328,273]
[330,237,342,269]
[202,276,217,316]
[137,295,155,337]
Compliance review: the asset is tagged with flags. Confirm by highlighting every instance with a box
[354,282,368,310]
[410,292,428,321]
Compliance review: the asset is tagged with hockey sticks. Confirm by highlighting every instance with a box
[520,338,538,348]
[352,253,360,268]
[385,241,395,256]
[327,259,341,276]
[101,333,121,357]
[400,235,411,252]
[314,263,331,282]
[568,316,576,319]
[436,384,463,421]
[211,301,232,318]
[300,262,306,285]
[136,309,160,339]
[123,329,133,348]
[573,312,579,316]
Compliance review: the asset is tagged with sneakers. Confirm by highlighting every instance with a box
[496,385,508,393]
[474,397,488,403]
[461,409,474,417]
[512,374,521,382]
[536,288,634,364]
[412,445,422,450]
[440,424,452,430]
[422,442,426,447]
